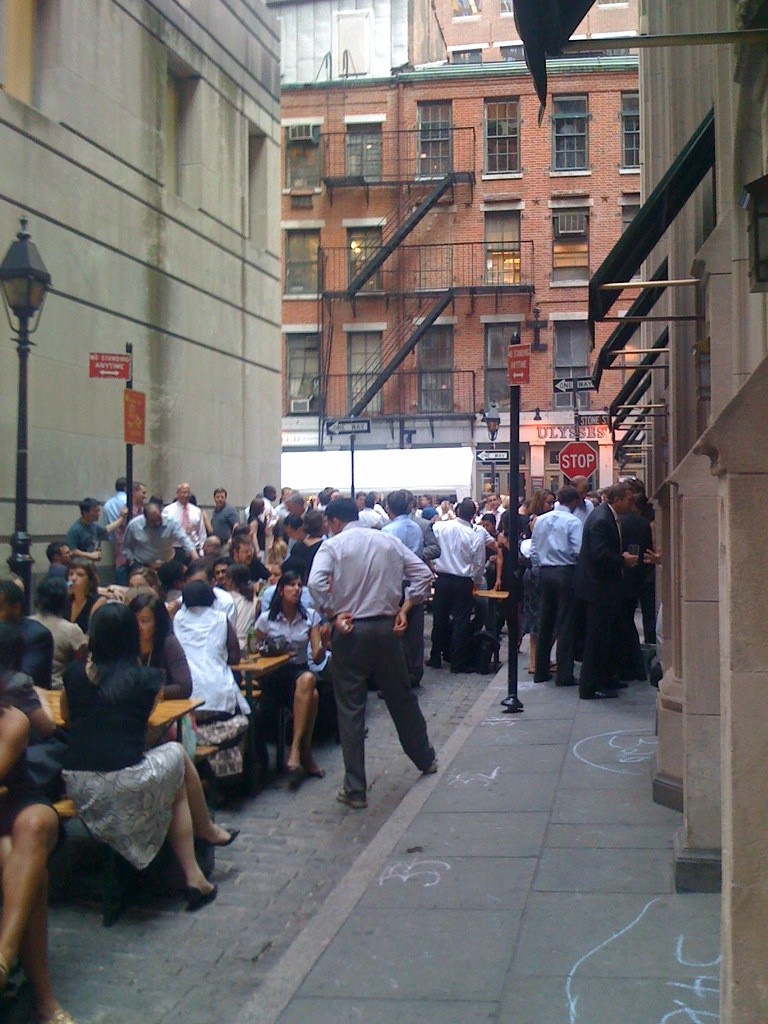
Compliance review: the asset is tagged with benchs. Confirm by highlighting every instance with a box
[230,654,291,777]
[49,795,128,927]
[191,743,219,804]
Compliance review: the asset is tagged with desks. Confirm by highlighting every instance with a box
[45,689,207,729]
[476,588,507,670]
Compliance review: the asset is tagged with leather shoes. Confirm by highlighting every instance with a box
[424,657,654,700]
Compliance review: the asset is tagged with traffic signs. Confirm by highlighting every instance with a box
[326,417,372,434]
[507,343,531,386]
[475,449,510,462]
[577,415,614,426]
[87,351,134,382]
[551,376,596,392]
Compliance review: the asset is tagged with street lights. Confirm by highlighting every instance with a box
[0,213,53,620]
[481,401,502,493]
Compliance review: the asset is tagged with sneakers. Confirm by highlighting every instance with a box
[424,755,439,773]
[336,788,369,809]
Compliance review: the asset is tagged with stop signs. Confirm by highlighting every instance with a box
[559,443,598,482]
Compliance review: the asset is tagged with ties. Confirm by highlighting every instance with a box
[182,504,189,534]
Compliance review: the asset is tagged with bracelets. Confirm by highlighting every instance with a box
[330,614,339,621]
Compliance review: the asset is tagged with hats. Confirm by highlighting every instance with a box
[421,507,439,519]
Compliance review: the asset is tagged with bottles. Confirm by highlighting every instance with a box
[247,623,258,654]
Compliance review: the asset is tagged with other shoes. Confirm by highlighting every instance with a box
[284,764,302,772]
[308,768,327,778]
[334,725,368,744]
[0,954,10,993]
[34,1004,80,1024]
[376,690,385,700]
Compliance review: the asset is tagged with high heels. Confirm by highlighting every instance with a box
[186,881,219,909]
[209,827,241,849]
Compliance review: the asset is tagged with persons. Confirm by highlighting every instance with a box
[0,478,659,1024]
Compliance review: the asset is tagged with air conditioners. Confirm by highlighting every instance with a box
[292,397,311,414]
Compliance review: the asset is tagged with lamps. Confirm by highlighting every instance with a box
[532,407,542,420]
[480,409,488,421]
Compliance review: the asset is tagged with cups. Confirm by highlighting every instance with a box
[628,544,639,556]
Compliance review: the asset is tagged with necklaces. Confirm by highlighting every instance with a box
[136,648,152,670]
